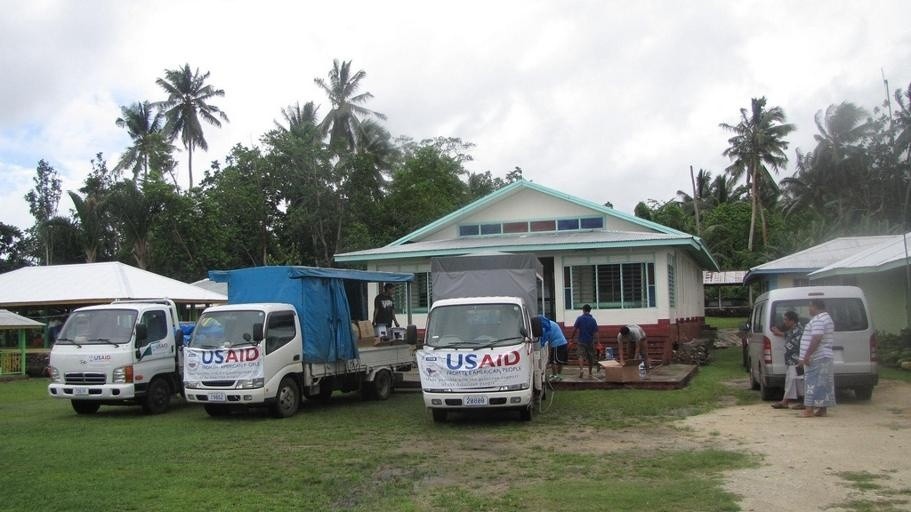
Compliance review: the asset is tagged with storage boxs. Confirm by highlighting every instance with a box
[597,359,644,382]
[356,320,374,339]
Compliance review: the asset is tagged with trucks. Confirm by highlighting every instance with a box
[48,296,225,414]
[417,249,546,424]
[184,265,417,418]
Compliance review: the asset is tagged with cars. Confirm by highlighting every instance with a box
[741,313,813,372]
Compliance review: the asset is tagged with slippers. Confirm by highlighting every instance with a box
[792,404,804,409]
[771,401,787,408]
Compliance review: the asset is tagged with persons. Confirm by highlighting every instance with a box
[796,298,835,417]
[569,304,600,379]
[371,283,399,338]
[616,323,649,374]
[543,320,568,383]
[770,310,804,411]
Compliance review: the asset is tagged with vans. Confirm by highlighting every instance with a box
[746,285,878,402]
